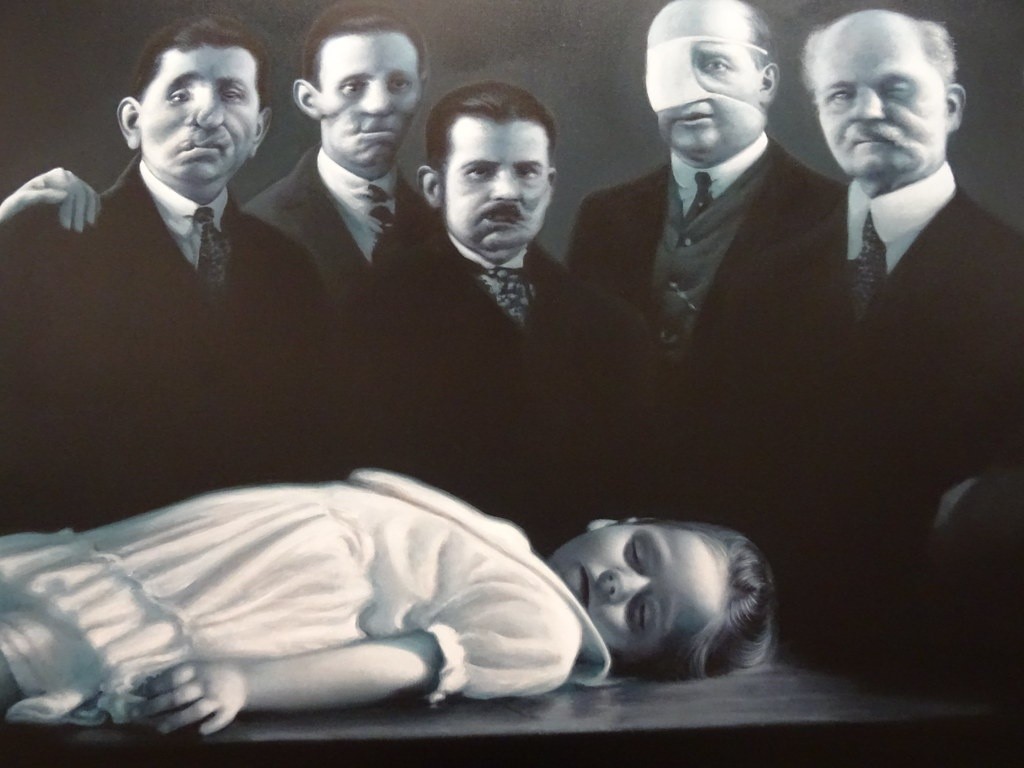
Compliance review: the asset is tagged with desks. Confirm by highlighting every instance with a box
[46,658,992,768]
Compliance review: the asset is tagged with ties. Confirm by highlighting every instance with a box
[193,207,230,296]
[369,186,396,261]
[484,266,531,326]
[850,212,888,307]
[684,172,713,223]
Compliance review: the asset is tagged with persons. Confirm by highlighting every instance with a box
[1,0,1023,767]
[0,466,774,738]
